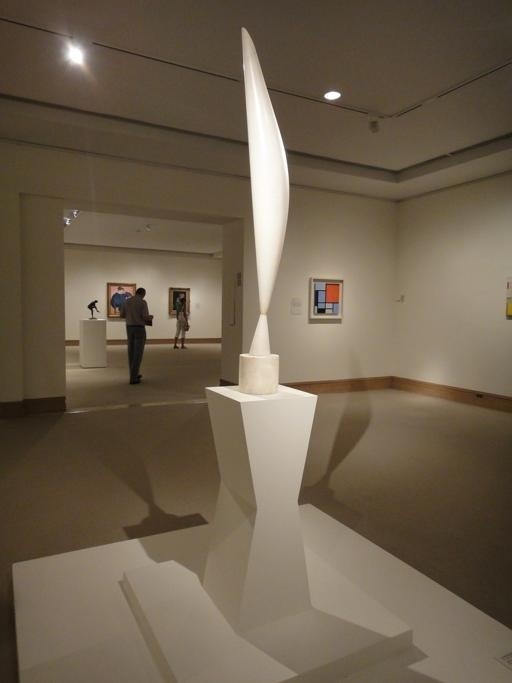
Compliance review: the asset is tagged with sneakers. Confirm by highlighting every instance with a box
[174,346,186,350]
[130,374,142,384]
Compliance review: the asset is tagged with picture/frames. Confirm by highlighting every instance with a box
[310,277,344,320]
[107,283,138,318]
[168,287,190,315]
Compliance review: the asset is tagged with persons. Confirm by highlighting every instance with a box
[173,298,189,349]
[119,288,153,384]
[87,300,99,319]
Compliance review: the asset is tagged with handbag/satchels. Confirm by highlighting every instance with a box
[184,321,189,331]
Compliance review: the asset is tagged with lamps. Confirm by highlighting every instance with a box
[72,207,80,222]
[368,110,380,134]
[64,217,71,228]
[143,224,151,233]
